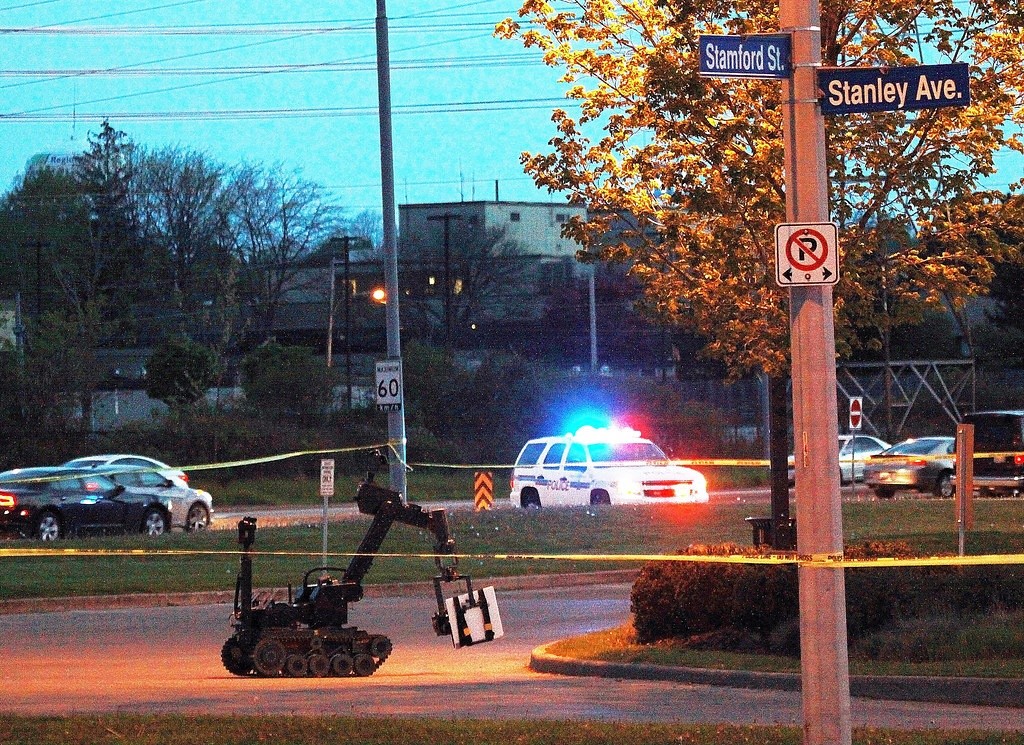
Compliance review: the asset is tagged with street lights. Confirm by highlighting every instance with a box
[327,287,385,366]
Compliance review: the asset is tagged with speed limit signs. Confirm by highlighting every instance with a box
[373,356,403,415]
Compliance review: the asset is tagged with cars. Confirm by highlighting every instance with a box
[788,435,893,486]
[95,465,215,534]
[0,467,173,542]
[862,434,956,498]
[59,454,189,487]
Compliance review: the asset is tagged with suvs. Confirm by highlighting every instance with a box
[509,425,711,507]
[952,410,1024,499]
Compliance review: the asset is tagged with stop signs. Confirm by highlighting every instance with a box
[848,396,863,431]
